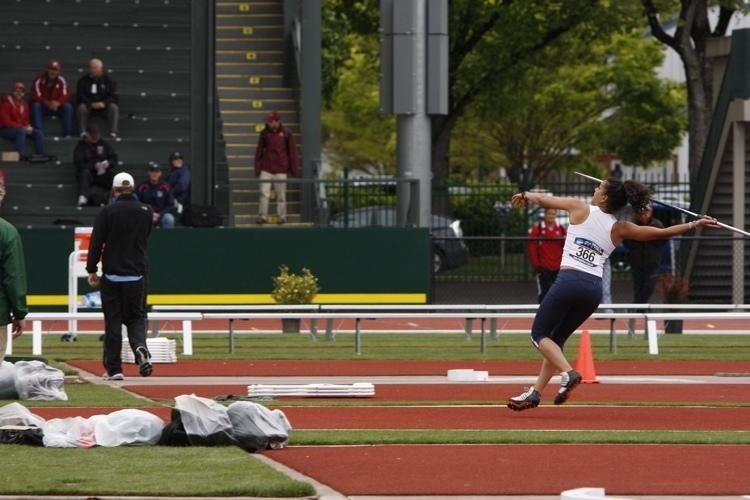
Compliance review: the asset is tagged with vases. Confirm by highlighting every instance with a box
[281,318,300,334]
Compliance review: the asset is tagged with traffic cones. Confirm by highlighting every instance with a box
[576,328,601,383]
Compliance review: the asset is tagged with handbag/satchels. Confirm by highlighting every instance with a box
[185,202,224,229]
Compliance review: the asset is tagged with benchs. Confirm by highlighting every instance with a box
[0,2,192,226]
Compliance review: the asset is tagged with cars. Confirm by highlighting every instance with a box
[337,176,473,197]
[329,207,470,274]
[526,186,691,272]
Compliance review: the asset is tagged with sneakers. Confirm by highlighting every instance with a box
[99,368,125,379]
[134,343,155,375]
[554,371,582,403]
[506,387,541,411]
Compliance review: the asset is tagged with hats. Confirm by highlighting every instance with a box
[265,110,280,122]
[111,172,136,189]
[171,151,184,160]
[12,80,26,91]
[149,161,160,172]
[48,59,59,71]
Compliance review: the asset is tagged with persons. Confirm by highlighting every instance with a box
[0,185,29,370]
[506,176,720,411]
[0,55,191,229]
[622,201,667,313]
[526,207,567,304]
[254,111,302,225]
[86,171,154,379]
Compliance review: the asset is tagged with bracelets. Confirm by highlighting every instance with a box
[521,191,527,201]
[692,220,696,230]
[689,222,691,232]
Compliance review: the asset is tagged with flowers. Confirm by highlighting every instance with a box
[273,264,320,305]
